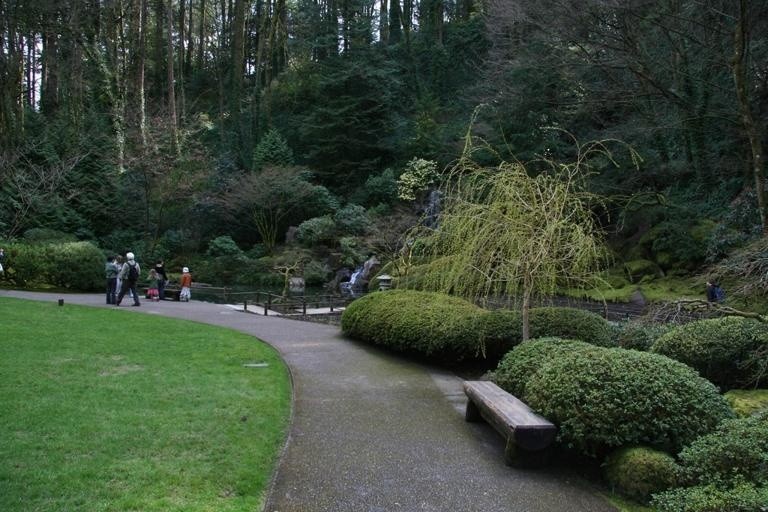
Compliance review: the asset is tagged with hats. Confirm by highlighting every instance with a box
[126,252,134,260]
[183,267,189,273]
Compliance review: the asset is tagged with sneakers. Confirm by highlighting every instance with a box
[132,303,140,306]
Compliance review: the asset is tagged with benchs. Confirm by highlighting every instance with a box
[142,287,181,301]
[462,379,557,470]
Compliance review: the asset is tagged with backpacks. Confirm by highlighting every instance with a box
[126,262,140,281]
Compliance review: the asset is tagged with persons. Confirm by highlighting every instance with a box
[180,267,191,301]
[155,260,169,300]
[146,269,160,301]
[707,281,719,302]
[105,256,118,304]
[114,256,124,293]
[115,252,141,306]
